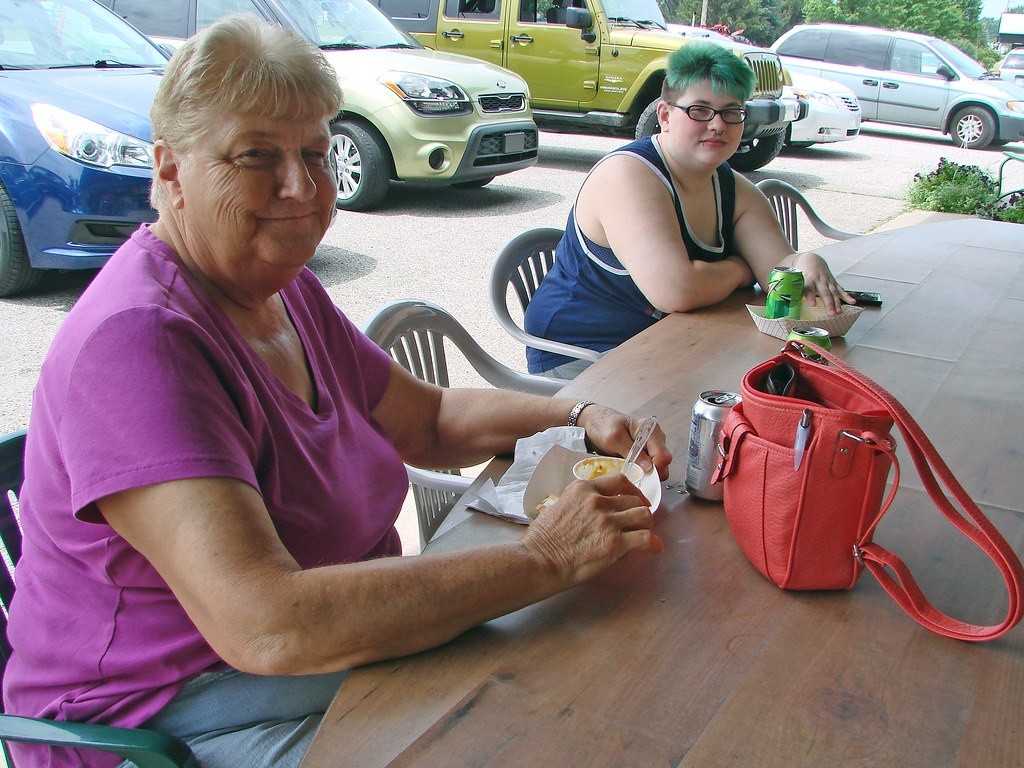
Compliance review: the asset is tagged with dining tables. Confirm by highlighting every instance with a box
[290,213,1024,768]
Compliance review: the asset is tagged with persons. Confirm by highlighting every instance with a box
[525,41,856,379]
[2,17,674,768]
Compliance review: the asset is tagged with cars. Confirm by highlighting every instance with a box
[0,1,173,297]
[665,25,863,150]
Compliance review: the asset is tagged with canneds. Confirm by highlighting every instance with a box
[765,266,804,320]
[786,326,831,366]
[685,389,743,504]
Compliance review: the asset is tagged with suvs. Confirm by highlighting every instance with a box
[995,45,1024,90]
[60,0,542,212]
[315,0,811,173]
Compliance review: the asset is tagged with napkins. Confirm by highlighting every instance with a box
[463,424,586,526]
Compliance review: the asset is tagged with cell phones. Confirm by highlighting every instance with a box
[839,291,882,305]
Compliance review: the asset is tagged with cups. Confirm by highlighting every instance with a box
[572,456,645,490]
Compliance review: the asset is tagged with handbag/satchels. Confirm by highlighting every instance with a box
[710,331,1024,642]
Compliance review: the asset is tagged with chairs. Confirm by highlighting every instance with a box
[477,0,494,12]
[360,296,570,554]
[0,428,200,768]
[756,180,867,249]
[547,0,573,25]
[490,225,607,361]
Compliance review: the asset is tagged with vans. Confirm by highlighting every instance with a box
[771,23,1024,150]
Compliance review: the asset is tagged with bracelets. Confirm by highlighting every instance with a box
[568,400,596,426]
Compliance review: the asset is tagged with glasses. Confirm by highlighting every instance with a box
[668,103,747,126]
